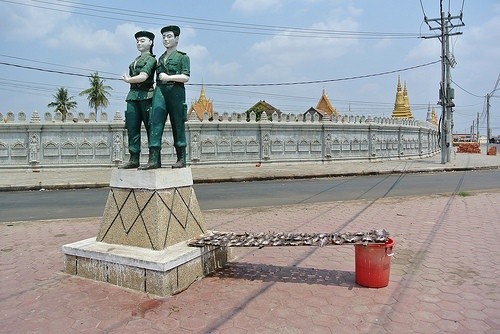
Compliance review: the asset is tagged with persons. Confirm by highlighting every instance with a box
[138,23,190,170]
[117,30,158,168]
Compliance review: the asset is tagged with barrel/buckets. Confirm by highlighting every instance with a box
[355,238,393,287]
